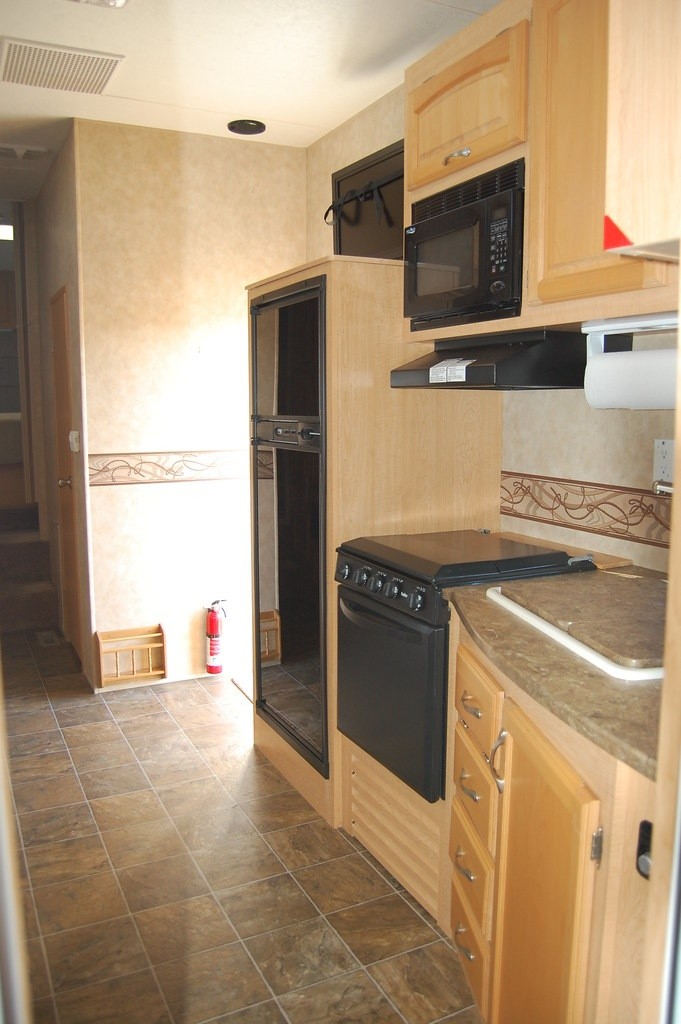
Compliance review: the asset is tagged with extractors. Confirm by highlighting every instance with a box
[391,330,588,392]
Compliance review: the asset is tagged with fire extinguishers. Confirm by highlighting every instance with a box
[206,598,229,674]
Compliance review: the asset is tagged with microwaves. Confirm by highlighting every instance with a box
[404,186,519,319]
